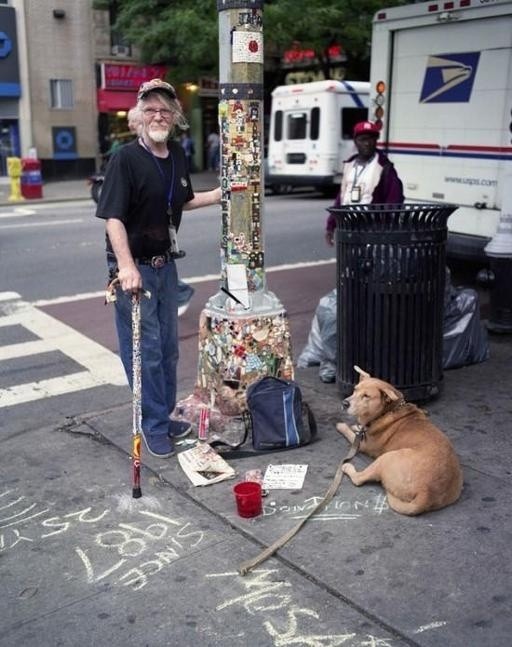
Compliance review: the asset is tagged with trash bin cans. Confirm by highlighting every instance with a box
[325,199,461,408]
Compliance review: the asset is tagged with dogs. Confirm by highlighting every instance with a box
[336,365,462,516]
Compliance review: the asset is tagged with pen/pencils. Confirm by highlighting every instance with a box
[219,285,246,308]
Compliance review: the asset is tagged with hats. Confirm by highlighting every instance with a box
[353,121,379,138]
[137,77,176,101]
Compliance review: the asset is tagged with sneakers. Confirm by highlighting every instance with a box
[169,420,192,438]
[141,428,175,458]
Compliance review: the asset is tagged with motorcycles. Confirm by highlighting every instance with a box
[87,172,105,203]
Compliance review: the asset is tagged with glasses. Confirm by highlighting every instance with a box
[142,108,172,116]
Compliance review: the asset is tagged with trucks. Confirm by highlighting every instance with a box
[365,0,512,287]
[266,80,370,195]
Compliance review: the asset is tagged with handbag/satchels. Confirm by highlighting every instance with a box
[211,375,317,460]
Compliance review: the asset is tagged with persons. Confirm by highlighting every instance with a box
[99,132,120,160]
[127,103,141,144]
[179,133,196,175]
[205,131,221,172]
[323,121,406,247]
[94,78,228,457]
